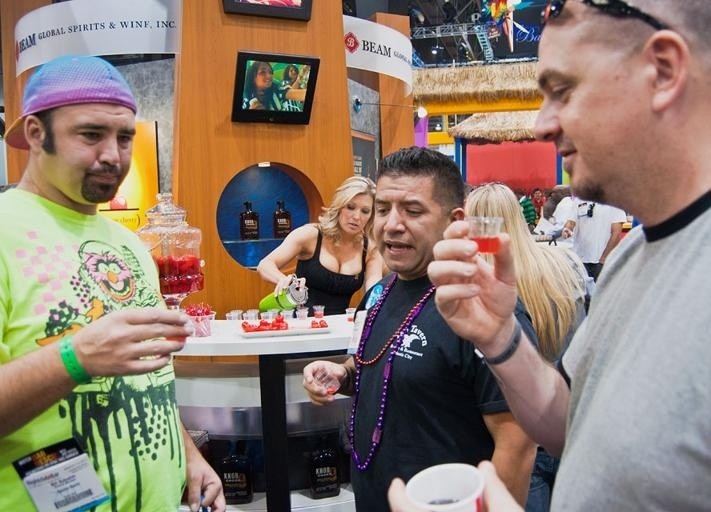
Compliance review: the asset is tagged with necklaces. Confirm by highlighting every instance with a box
[354,270,436,366]
[349,270,437,471]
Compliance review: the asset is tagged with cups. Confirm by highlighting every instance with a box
[313,367,342,395]
[296,307,309,321]
[405,462,486,511]
[187,309,218,339]
[260,309,279,321]
[280,308,295,321]
[226,309,243,321]
[465,216,505,254]
[313,305,326,318]
[346,308,357,322]
[165,305,186,341]
[243,309,259,321]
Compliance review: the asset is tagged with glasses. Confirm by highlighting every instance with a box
[588,202,595,217]
[540,0,669,30]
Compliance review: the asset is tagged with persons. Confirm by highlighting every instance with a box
[257,175,385,316]
[465,178,638,510]
[302,146,537,512]
[279,65,311,89]
[243,60,306,111]
[388,0,711,511]
[0,54,228,511]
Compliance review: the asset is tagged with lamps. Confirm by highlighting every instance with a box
[353,97,426,118]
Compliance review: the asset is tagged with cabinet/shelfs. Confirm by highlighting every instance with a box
[222,237,288,270]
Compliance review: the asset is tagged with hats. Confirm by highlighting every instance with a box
[4,54,137,149]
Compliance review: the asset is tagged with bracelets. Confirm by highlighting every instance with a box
[57,335,93,386]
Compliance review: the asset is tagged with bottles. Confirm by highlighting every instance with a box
[241,201,260,240]
[273,200,292,239]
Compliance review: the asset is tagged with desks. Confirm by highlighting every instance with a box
[167,311,370,510]
